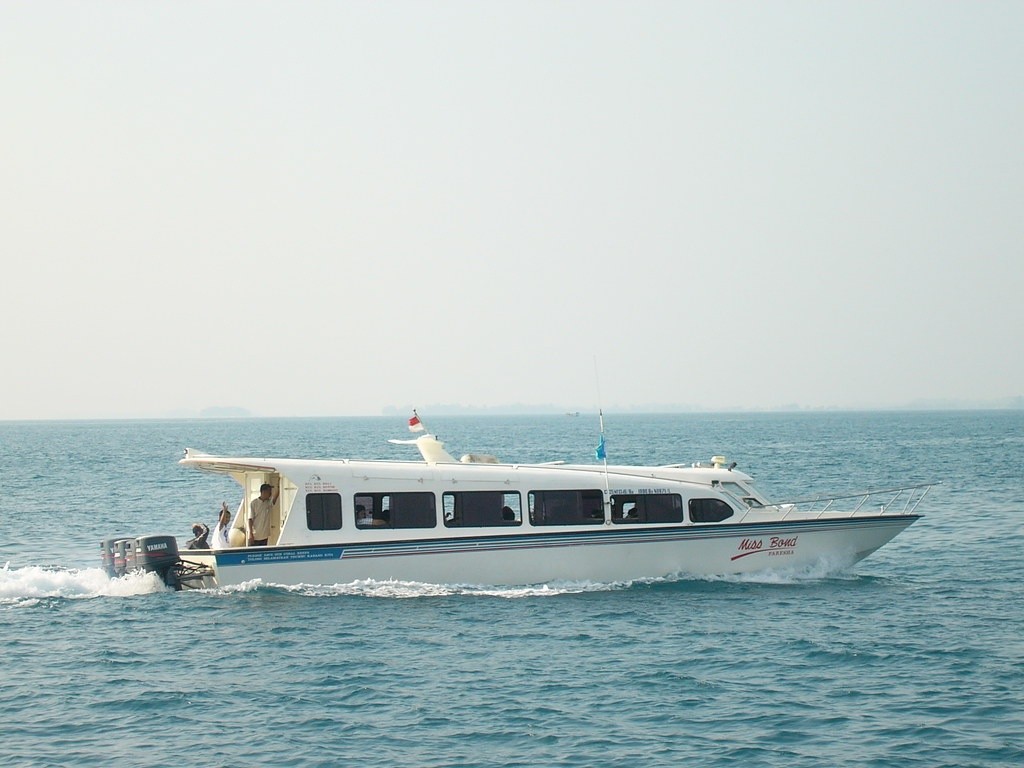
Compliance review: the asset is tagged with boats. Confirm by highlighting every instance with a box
[98,410,928,590]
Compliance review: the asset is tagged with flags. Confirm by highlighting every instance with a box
[405,416,424,433]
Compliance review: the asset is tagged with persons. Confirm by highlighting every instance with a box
[211,500,230,549]
[184,521,210,549]
[248,477,280,546]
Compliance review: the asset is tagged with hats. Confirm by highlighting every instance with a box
[503,506,515,520]
[260,483,274,492]
[355,505,365,514]
